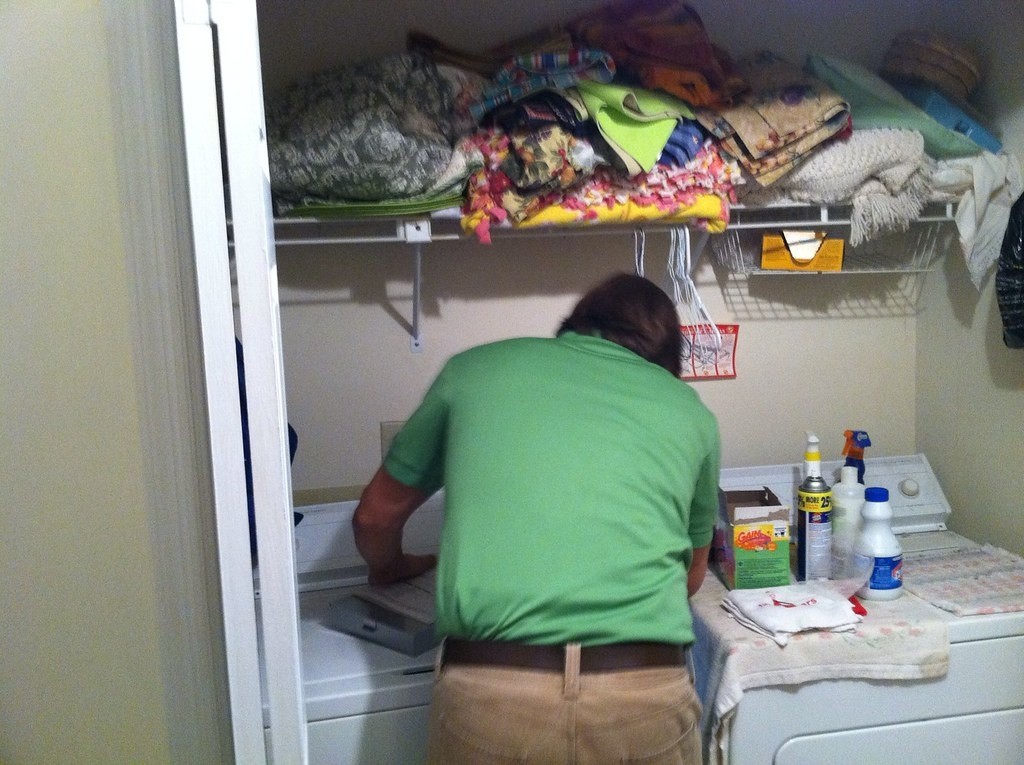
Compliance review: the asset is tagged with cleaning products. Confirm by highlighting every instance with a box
[795,427,906,602]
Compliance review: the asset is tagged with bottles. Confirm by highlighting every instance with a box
[798,470,832,581]
[831,466,865,579]
[854,488,902,600]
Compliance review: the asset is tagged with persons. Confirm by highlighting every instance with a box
[351,274,721,765]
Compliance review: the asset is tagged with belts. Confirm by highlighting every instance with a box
[440,636,690,672]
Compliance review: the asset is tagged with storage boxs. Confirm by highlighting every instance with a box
[715,485,791,591]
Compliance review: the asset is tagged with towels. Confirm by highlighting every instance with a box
[719,577,865,649]
[688,550,950,765]
